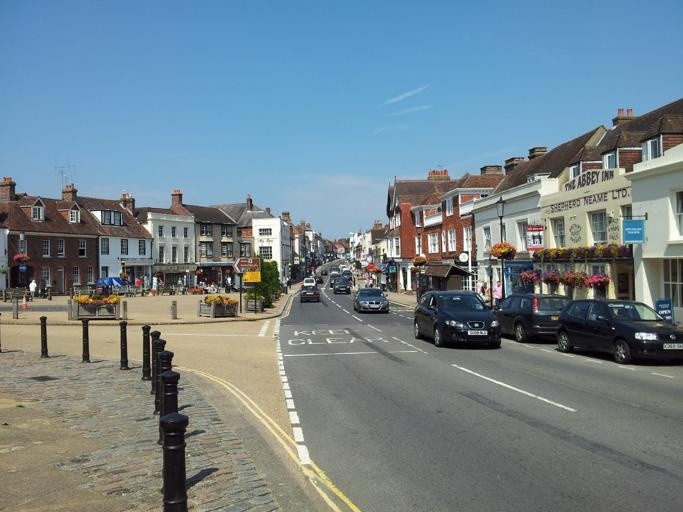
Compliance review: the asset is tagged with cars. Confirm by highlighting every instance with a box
[413,288,683,367]
[300,264,389,316]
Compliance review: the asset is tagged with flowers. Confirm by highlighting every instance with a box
[413,256,428,268]
[11,253,31,264]
[201,294,239,312]
[519,240,630,291]
[491,240,516,262]
[71,293,121,314]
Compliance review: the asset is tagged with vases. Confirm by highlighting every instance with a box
[17,262,27,273]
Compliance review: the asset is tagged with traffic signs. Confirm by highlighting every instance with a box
[233,258,260,273]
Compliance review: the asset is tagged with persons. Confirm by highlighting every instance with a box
[479,282,490,298]
[362,271,377,288]
[29,280,37,301]
[492,281,503,307]
[135,277,141,287]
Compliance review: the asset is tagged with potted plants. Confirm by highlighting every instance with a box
[243,292,267,313]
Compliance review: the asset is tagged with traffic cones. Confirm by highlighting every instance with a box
[21,295,27,310]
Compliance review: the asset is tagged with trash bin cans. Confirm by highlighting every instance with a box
[73,283,81,296]
[86,282,96,294]
[225,285,230,293]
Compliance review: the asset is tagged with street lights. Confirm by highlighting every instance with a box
[496,197,507,299]
